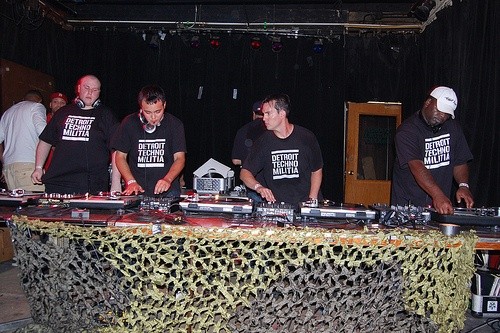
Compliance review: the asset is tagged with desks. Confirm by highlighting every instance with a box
[0,202,500,251]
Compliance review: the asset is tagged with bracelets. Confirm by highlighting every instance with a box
[459,182,470,189]
[36,166,42,169]
[254,183,262,190]
[128,180,137,185]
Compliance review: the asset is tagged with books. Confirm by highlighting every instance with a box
[473,271,500,298]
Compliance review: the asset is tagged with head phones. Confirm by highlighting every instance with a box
[74,94,101,107]
[138,113,165,125]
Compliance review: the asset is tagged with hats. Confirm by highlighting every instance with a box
[49,91,67,103]
[252,96,272,111]
[430,86,458,119]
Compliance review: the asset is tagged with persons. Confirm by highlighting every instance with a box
[44,89,71,196]
[233,102,269,189]
[239,93,326,206]
[32,74,124,197]
[0,90,50,264]
[112,85,188,198]
[391,87,476,214]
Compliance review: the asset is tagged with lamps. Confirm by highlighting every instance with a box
[191,35,200,48]
[310,38,327,53]
[248,35,263,51]
[208,34,222,49]
[270,36,283,53]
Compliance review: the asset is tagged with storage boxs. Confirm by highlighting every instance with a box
[466,293,500,318]
